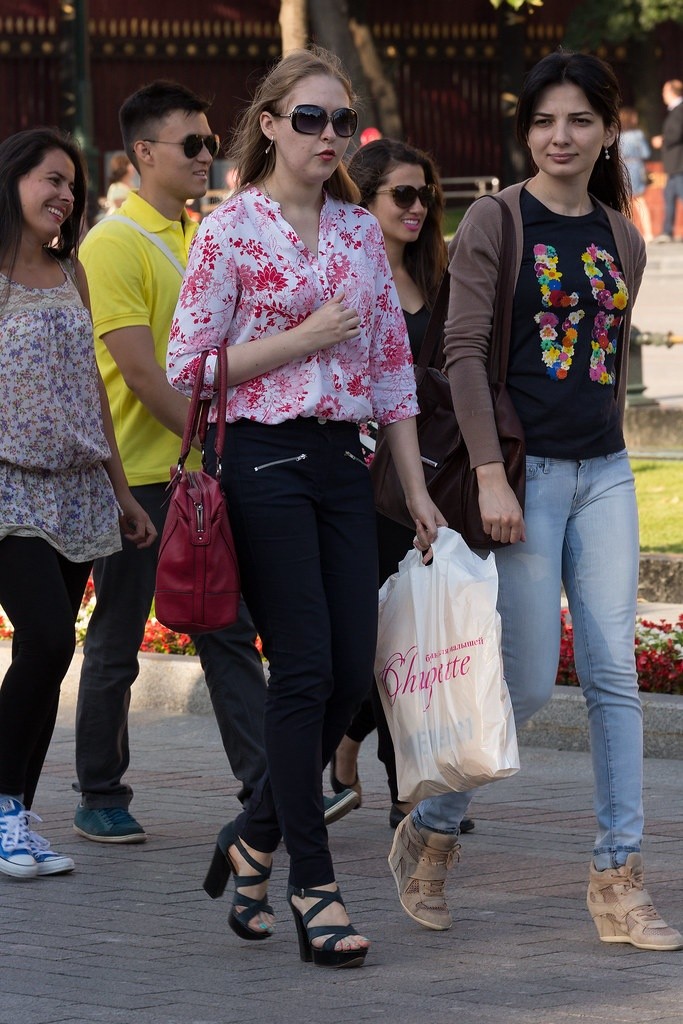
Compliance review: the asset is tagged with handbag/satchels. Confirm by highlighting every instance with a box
[154,343,243,637]
[373,527,520,803]
[367,195,527,551]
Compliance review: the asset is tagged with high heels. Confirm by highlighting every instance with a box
[287,884,369,968]
[587,852,683,950]
[202,820,276,941]
[387,813,462,930]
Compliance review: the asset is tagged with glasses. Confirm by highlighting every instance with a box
[133,134,221,159]
[271,104,359,138]
[372,184,437,208]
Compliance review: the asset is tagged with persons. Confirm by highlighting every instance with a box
[328,138,475,834]
[107,153,135,216]
[71,86,362,843]
[164,52,448,970]
[618,107,655,242]
[388,52,683,953]
[650,78,683,242]
[0,130,157,882]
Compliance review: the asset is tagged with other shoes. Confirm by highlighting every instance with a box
[330,751,362,810]
[390,804,476,832]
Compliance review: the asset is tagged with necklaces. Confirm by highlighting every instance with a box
[262,182,271,200]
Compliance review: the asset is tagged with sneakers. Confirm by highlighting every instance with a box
[323,789,359,825]
[0,797,43,878]
[73,802,148,844]
[29,830,75,875]
